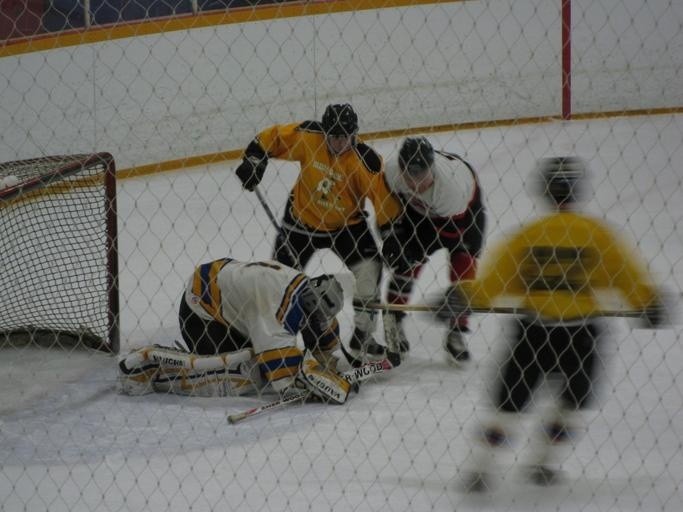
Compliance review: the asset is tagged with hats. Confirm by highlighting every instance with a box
[399,136,434,171]
[322,103,358,136]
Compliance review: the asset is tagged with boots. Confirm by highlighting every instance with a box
[442,327,470,366]
[350,308,408,359]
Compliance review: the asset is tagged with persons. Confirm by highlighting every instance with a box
[233,101,407,361]
[117,257,351,407]
[379,131,486,361]
[426,152,667,496]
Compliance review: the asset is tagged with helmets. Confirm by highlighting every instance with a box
[534,156,587,201]
[304,274,343,318]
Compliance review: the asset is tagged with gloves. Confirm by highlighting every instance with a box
[382,232,403,267]
[236,139,269,192]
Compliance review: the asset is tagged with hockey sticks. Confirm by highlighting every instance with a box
[227,310,401,423]
[354,261,389,393]
[253,184,366,367]
